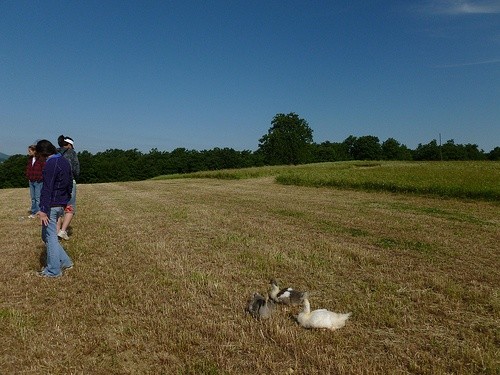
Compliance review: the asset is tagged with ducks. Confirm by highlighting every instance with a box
[295,298,352,330]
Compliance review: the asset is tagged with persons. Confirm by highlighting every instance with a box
[27,144,45,214]
[33,139,74,278]
[54,135,76,241]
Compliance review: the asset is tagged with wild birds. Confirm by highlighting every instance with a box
[245,292,274,320]
[268,280,304,307]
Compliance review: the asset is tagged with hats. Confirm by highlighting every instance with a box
[64,139,75,149]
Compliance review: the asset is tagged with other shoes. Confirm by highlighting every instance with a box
[36,262,73,278]
[28,214,39,218]
[57,229,69,240]
